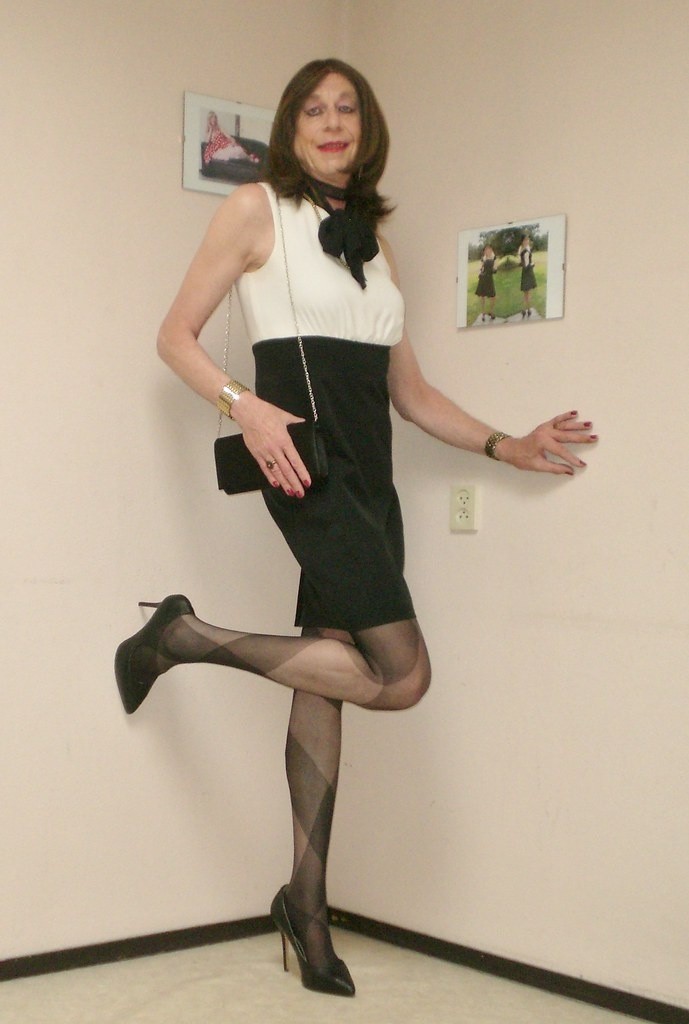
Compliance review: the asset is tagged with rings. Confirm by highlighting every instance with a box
[266,459,276,469]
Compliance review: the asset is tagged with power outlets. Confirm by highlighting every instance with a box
[449,484,479,531]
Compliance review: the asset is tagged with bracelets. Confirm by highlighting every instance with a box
[485,432,512,461]
[217,380,251,420]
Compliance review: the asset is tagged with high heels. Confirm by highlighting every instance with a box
[271,884,356,997]
[114,595,196,714]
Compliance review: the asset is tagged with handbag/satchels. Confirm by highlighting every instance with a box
[214,421,331,495]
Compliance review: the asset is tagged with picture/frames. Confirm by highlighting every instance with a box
[184,89,277,197]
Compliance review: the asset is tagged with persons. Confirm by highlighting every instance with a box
[518,234,537,318]
[204,110,259,164]
[115,59,600,996]
[475,243,498,322]
